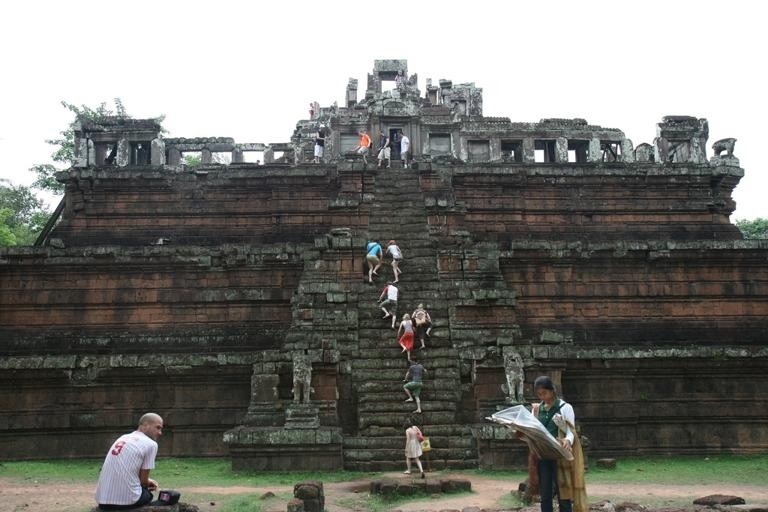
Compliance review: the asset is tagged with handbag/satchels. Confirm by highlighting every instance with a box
[526,446,541,496]
[419,437,432,451]
[415,425,425,442]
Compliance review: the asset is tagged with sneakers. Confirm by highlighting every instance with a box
[420,472,425,478]
[403,470,411,474]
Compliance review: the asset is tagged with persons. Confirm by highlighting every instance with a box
[352,130,372,168]
[384,239,404,283]
[396,129,411,170]
[411,303,433,349]
[94,411,164,512]
[514,376,577,512]
[314,126,328,164]
[377,280,400,331]
[366,238,384,284]
[377,130,393,170]
[403,355,427,414]
[396,313,417,363]
[403,416,426,479]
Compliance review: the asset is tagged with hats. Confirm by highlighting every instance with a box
[149,489,181,506]
